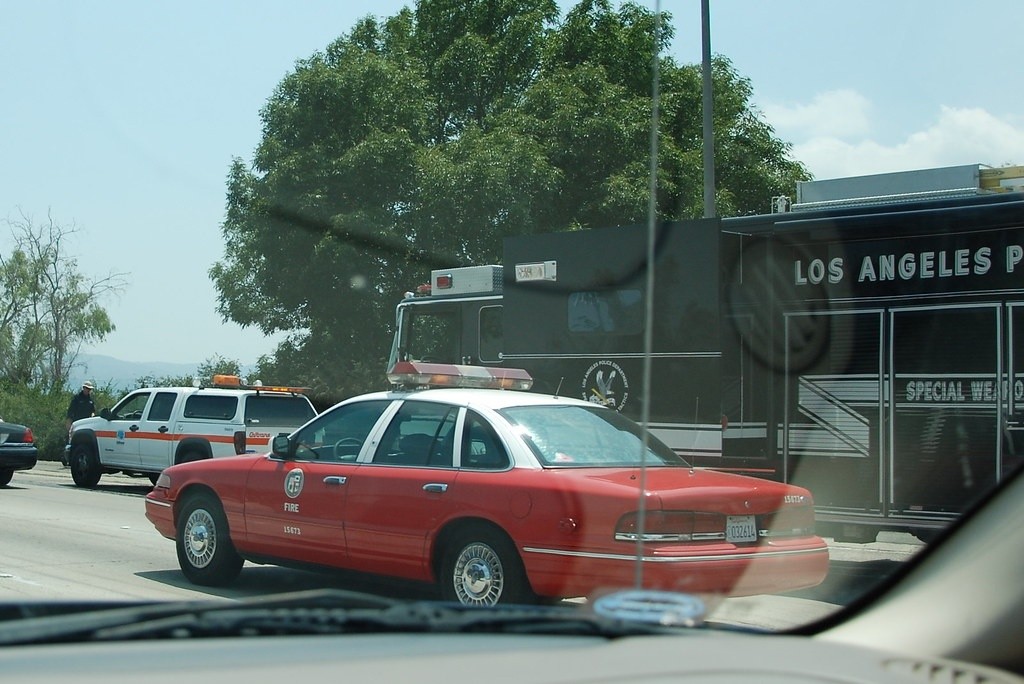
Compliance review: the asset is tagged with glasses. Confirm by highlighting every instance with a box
[86,388,91,390]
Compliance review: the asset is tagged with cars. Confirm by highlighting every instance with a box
[0,418,40,488]
[144,363,831,611]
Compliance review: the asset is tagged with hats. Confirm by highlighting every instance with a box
[83,381,94,388]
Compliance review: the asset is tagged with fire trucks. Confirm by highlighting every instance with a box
[385,163,1024,543]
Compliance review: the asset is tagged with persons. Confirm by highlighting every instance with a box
[60,381,95,466]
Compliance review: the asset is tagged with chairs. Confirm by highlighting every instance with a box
[393,433,441,466]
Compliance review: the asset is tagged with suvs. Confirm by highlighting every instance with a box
[59,375,326,487]
